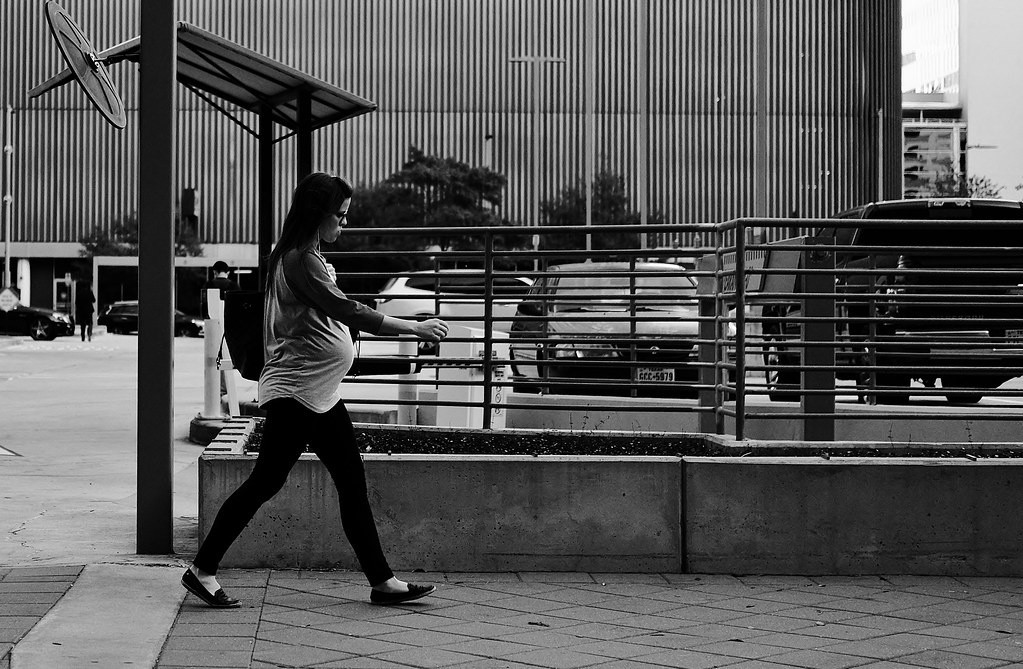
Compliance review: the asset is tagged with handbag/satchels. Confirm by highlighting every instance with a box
[216,286,376,381]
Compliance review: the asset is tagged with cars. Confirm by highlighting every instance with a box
[507,260,735,394]
[105,299,205,337]
[0,303,76,342]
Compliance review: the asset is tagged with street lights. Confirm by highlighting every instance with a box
[510,55,567,272]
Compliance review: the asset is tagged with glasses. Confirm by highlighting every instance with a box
[334,209,352,227]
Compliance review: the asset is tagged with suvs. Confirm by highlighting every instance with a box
[761,198,1023,406]
[371,268,534,333]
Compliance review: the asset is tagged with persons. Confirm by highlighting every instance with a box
[75,277,96,342]
[202,260,240,414]
[180,172,450,609]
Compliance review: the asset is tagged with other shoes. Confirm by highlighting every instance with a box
[183,570,243,609]
[370,581,437,604]
